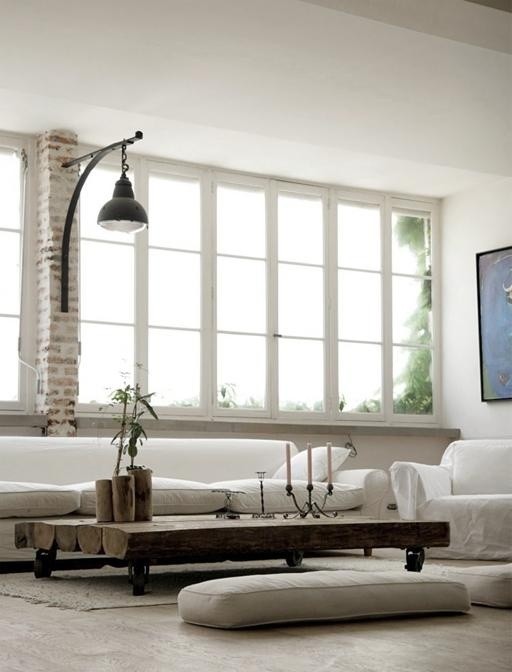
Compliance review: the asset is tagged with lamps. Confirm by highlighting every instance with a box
[59,130,147,312]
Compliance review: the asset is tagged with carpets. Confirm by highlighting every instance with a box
[0,555,319,613]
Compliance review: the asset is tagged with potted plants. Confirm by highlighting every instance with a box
[95,363,153,521]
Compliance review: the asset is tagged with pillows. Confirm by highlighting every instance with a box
[453,445,512,494]
[450,566,512,609]
[271,447,351,482]
[178,570,470,630]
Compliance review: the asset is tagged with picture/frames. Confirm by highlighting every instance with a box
[476,245,512,402]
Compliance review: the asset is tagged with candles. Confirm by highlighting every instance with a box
[285,444,291,483]
[308,442,313,484]
[327,442,333,484]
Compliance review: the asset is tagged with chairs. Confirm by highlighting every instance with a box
[388,439,512,561]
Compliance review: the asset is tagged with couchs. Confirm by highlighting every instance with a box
[0,436,390,562]
